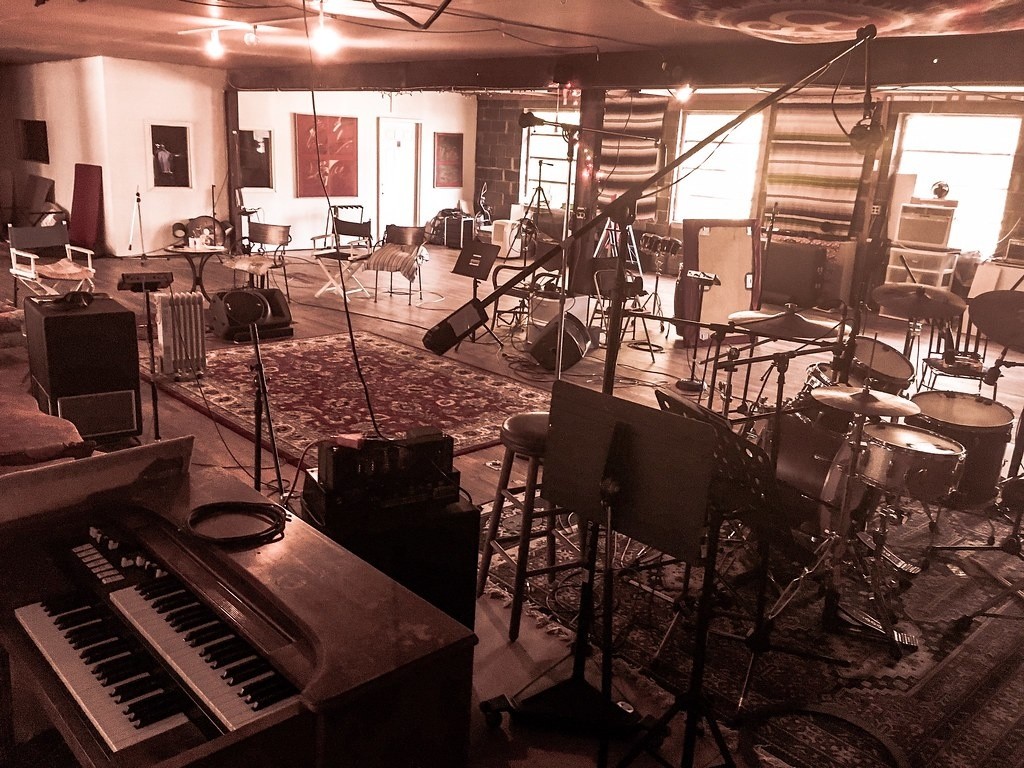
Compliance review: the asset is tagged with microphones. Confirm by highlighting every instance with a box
[852,125,885,150]
[655,144,667,186]
[944,327,955,365]
[984,347,1009,386]
[839,321,859,384]
[830,306,847,382]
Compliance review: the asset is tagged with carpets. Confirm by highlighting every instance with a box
[476,476,1024,767]
[137,327,552,472]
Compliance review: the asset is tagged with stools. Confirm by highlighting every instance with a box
[475,411,601,640]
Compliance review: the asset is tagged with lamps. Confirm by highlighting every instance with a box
[244,24,261,45]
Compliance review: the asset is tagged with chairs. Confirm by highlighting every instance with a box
[232,222,292,304]
[7,220,96,308]
[361,225,427,306]
[916,297,987,397]
[310,218,374,302]
[0,199,65,248]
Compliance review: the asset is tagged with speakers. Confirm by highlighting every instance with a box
[528,313,591,372]
[23,292,143,440]
[208,289,292,340]
[492,220,521,258]
[301,468,480,768]
[444,217,474,250]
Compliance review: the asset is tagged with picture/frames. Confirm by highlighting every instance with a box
[145,119,194,190]
[432,133,464,190]
[237,129,277,193]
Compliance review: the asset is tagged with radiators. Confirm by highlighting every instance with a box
[154,291,207,382]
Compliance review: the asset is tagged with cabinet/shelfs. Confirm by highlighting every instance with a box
[526,293,589,344]
[879,248,959,324]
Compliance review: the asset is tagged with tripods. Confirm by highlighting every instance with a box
[502,159,562,266]
[616,285,1024,768]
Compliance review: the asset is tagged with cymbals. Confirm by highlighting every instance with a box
[871,282,967,318]
[809,386,922,418]
[968,288,1024,354]
[727,309,854,340]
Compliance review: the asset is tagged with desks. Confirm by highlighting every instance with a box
[164,246,227,303]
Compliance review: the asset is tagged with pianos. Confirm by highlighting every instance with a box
[0,463,481,767]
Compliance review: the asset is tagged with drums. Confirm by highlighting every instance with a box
[856,421,968,501]
[907,389,1016,507]
[788,362,866,440]
[839,335,915,395]
[754,396,867,542]
[639,232,682,258]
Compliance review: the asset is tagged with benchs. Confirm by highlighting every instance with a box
[0,302,105,477]
